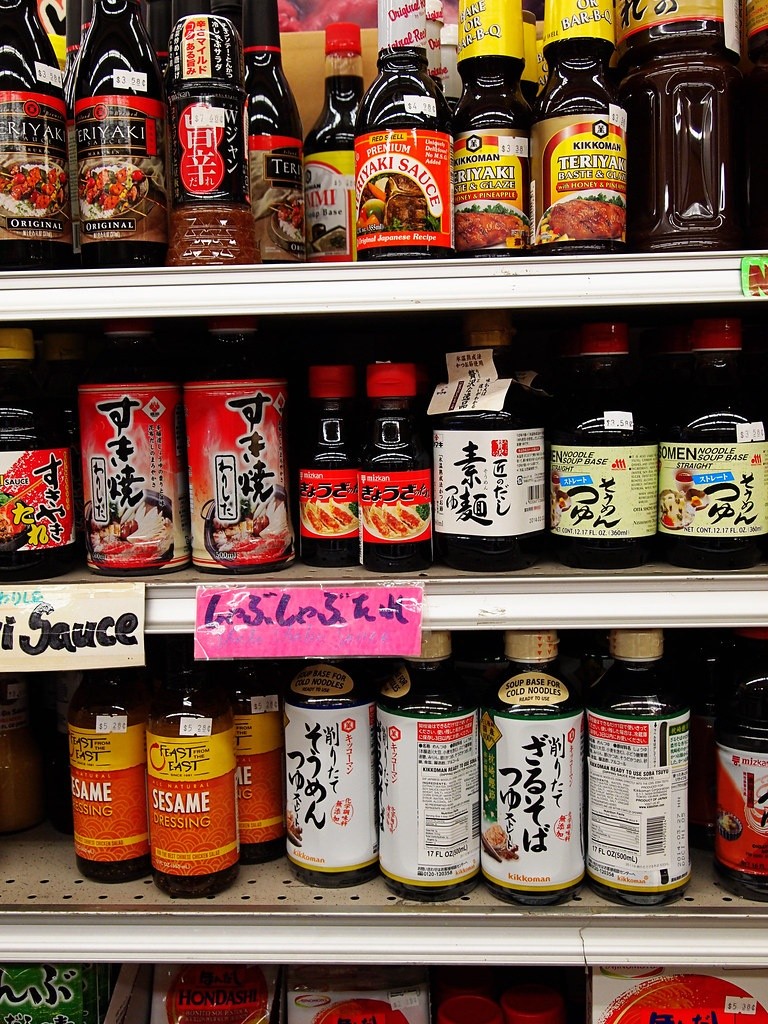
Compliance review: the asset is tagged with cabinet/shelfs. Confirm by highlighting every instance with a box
[1,244,768,1024]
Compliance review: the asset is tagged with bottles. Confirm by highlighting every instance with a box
[240,1,303,264]
[479,626,768,906]
[1,326,90,581]
[0,671,109,832]
[296,362,433,572]
[61,633,151,884]
[429,972,570,1024]
[142,634,240,898]
[302,0,539,261]
[231,659,289,865]
[378,634,482,904]
[0,0,255,271]
[549,321,661,570]
[182,316,300,575]
[431,308,554,572]
[77,318,192,577]
[531,0,768,254]
[657,317,768,570]
[280,659,379,889]
